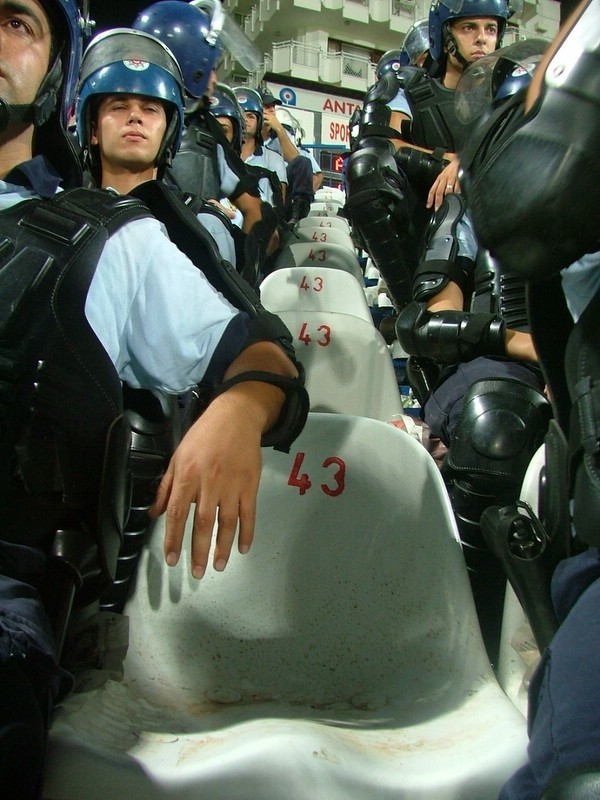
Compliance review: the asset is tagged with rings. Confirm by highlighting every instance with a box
[446,184,454,189]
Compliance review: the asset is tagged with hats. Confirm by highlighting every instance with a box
[256,88,283,106]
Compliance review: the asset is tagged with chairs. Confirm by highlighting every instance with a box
[46,185,548,800]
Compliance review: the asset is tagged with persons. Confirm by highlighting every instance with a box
[74,0,324,607]
[0,0,310,800]
[341,0,600,800]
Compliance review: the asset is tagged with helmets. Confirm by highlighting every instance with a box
[76,28,184,158]
[209,82,247,152]
[375,50,402,80]
[231,87,264,131]
[428,0,509,60]
[132,0,220,99]
[490,62,543,111]
[31,0,83,125]
[399,19,431,65]
